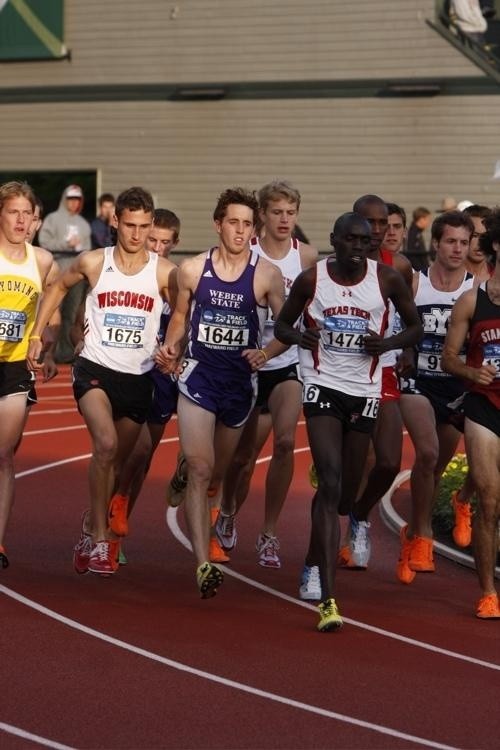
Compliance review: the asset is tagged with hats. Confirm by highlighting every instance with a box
[456,201,474,212]
[435,198,456,211]
[67,190,81,197]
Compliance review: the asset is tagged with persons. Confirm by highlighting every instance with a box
[1,179,500,633]
